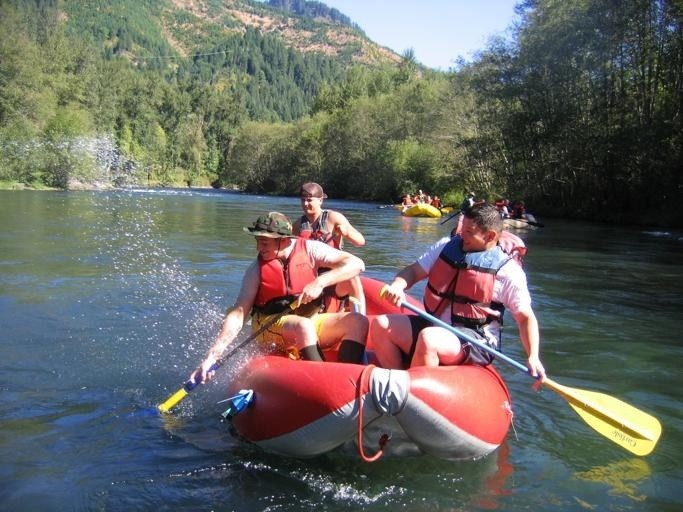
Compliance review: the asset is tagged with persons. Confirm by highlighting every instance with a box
[291,182,366,316]
[371,203,546,392]
[187,211,370,385]
[402,190,442,209]
[460,191,527,219]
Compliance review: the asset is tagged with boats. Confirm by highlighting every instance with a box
[226,277,512,464]
[641,229,682,241]
[401,203,441,218]
[399,215,442,235]
[502,214,538,229]
[489,318,490,321]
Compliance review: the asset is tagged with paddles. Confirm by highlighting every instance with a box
[440,206,453,214]
[510,217,544,228]
[381,285,661,456]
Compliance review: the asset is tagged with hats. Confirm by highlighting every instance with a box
[243,211,298,240]
[299,183,328,199]
